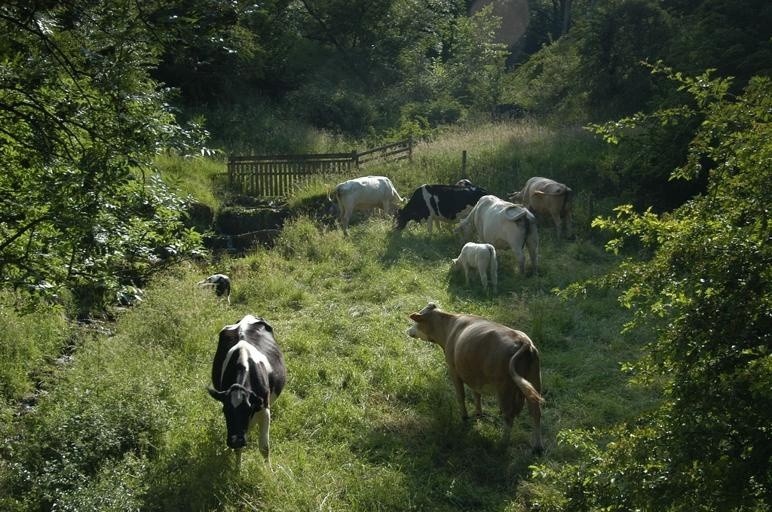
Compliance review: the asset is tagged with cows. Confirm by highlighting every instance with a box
[395,179,491,234]
[451,242,498,297]
[196,274,231,306]
[324,175,409,237]
[506,176,574,241]
[454,194,540,276]
[405,301,547,458]
[206,314,287,471]
